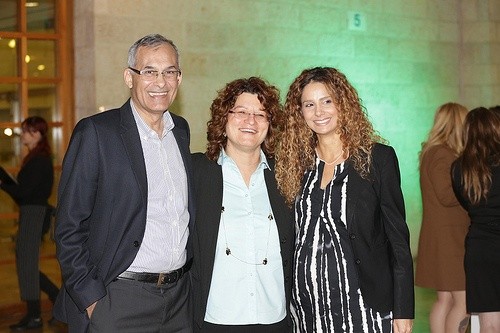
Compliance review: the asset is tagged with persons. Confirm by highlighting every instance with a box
[274,66,415,333]
[10,116,61,333]
[414,101,469,333]
[190,76,292,333]
[53,34,197,333]
[450,107,500,333]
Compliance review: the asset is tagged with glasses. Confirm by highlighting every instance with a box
[127,66,181,79]
[227,109,269,122]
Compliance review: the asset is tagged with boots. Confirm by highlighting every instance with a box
[46,284,62,324]
[8,299,42,332]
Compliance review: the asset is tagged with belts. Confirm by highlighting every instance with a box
[115,257,194,285]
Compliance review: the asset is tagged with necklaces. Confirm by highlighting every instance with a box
[220,205,273,266]
